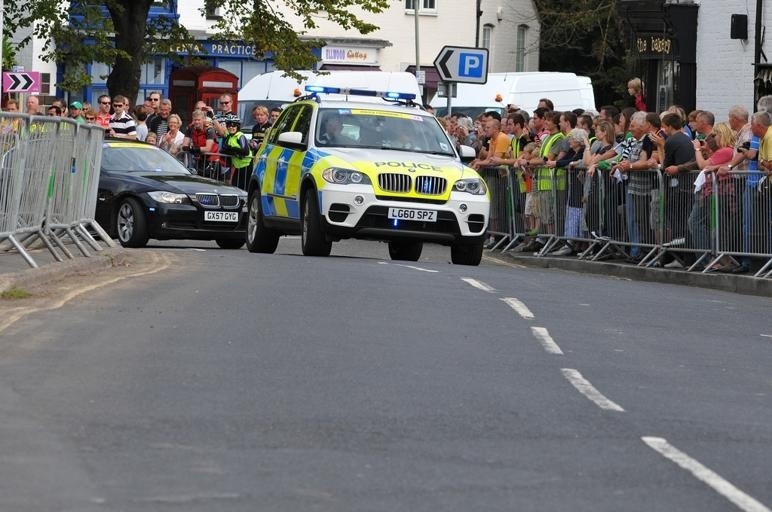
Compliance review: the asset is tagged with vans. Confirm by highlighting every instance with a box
[428,71,596,134]
[237,70,423,156]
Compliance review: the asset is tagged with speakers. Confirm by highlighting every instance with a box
[730,13,748,40]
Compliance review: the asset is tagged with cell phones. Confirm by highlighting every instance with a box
[543,156,548,161]
[105,129,111,133]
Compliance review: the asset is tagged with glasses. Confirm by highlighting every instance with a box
[114,105,122,108]
[228,123,237,127]
[149,99,158,101]
[220,102,229,104]
[102,102,111,105]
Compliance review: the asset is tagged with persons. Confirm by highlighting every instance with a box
[422,95,772,272]
[325,115,358,145]
[0,90,284,192]
[628,77,646,110]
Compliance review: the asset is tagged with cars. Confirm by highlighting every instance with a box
[1,136,252,249]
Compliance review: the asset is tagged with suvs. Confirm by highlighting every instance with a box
[248,86,490,266]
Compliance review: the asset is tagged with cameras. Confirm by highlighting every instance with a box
[468,125,479,134]
[699,140,705,146]
[202,107,208,111]
[737,142,750,153]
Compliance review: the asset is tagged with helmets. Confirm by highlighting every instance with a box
[225,113,240,124]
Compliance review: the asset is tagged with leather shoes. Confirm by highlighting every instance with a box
[521,240,534,252]
[530,243,544,252]
[732,266,749,273]
[487,240,498,249]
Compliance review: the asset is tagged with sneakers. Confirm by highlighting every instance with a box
[663,259,683,268]
[652,260,661,267]
[528,229,537,236]
[632,255,643,264]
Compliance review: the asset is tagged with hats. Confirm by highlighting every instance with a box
[70,101,83,110]
